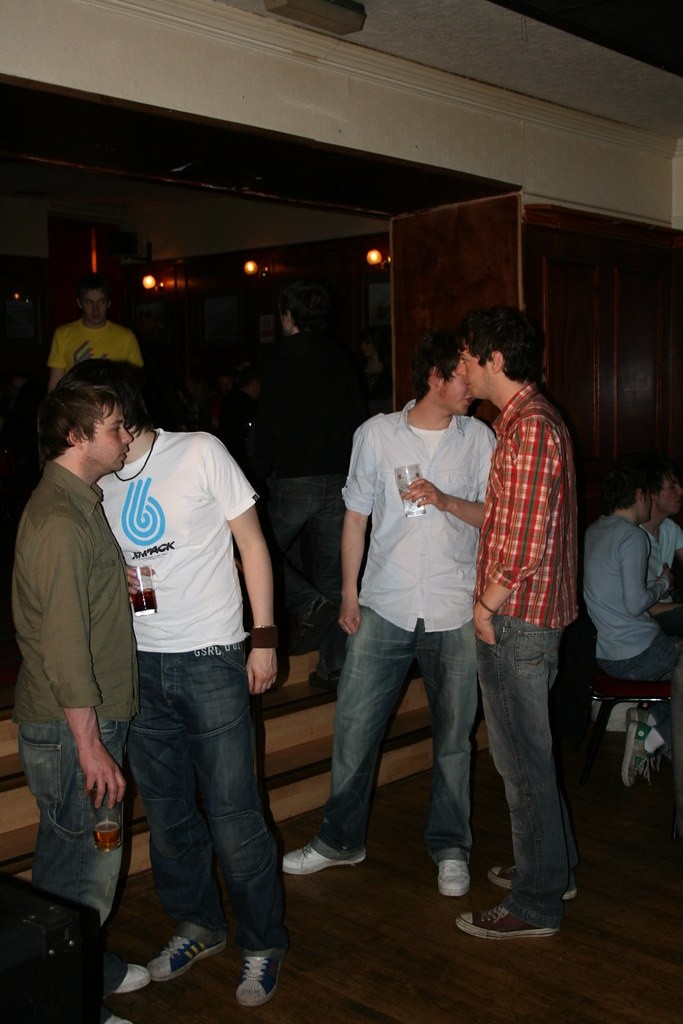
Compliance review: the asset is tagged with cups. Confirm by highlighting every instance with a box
[394,464,426,518]
[131,565,158,617]
[90,788,121,852]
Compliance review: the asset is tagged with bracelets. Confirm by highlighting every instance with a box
[478,597,491,612]
[250,625,278,648]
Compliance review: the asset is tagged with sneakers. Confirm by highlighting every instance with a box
[105,1014,132,1024]
[236,954,283,1005]
[438,857,470,896]
[627,707,650,733]
[487,867,577,899]
[114,963,151,992]
[282,844,366,875]
[622,720,658,786]
[455,906,559,939]
[148,935,228,980]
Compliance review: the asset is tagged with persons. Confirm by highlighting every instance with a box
[359,323,393,414]
[253,282,361,690]
[454,306,582,937]
[578,453,683,786]
[10,381,152,1024]
[60,359,292,1005]
[46,272,144,394]
[282,327,496,895]
[138,352,265,490]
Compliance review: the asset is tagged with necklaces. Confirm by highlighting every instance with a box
[115,429,157,481]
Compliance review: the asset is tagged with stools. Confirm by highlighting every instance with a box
[578,671,672,787]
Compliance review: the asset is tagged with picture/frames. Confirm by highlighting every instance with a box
[194,284,245,348]
[358,262,390,357]
[137,296,174,346]
